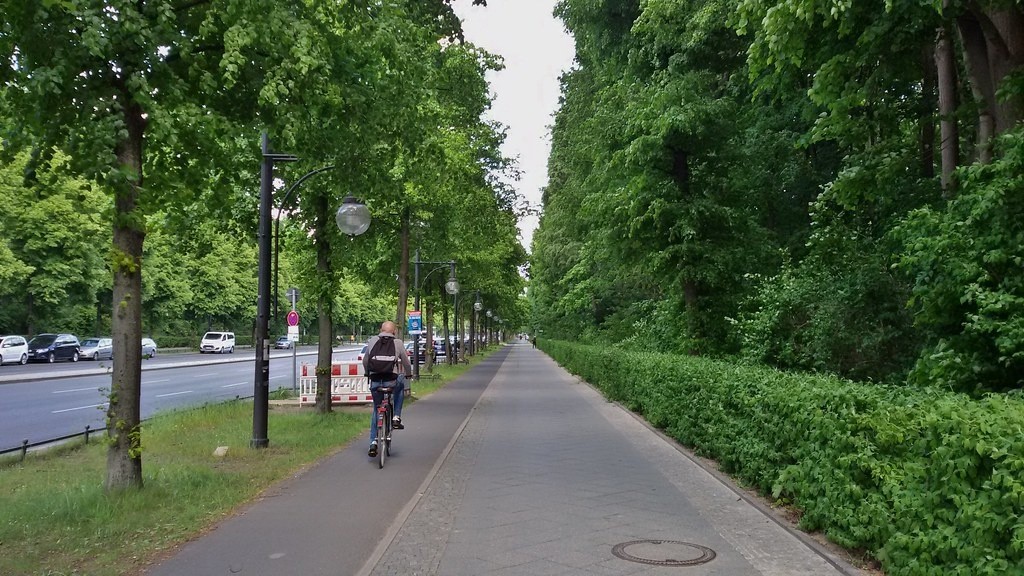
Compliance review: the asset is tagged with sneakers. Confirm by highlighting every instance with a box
[392,417,404,429]
[369,445,378,456]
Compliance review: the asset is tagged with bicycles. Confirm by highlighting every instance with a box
[364,374,413,468]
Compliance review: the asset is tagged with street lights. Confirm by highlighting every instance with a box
[453,288,502,365]
[411,250,459,381]
[249,134,371,451]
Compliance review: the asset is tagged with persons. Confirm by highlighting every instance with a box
[362,321,412,457]
[523,332,526,340]
[351,334,354,341]
[335,334,341,341]
[526,335,529,343]
[518,333,523,340]
[532,335,536,349]
[341,334,345,346]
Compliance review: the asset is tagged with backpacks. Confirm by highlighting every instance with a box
[368,336,400,375]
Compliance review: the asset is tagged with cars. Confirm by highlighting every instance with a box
[274,336,294,351]
[404,338,482,365]
[0,332,113,365]
[141,338,156,358]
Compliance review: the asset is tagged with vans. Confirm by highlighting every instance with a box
[199,331,236,355]
